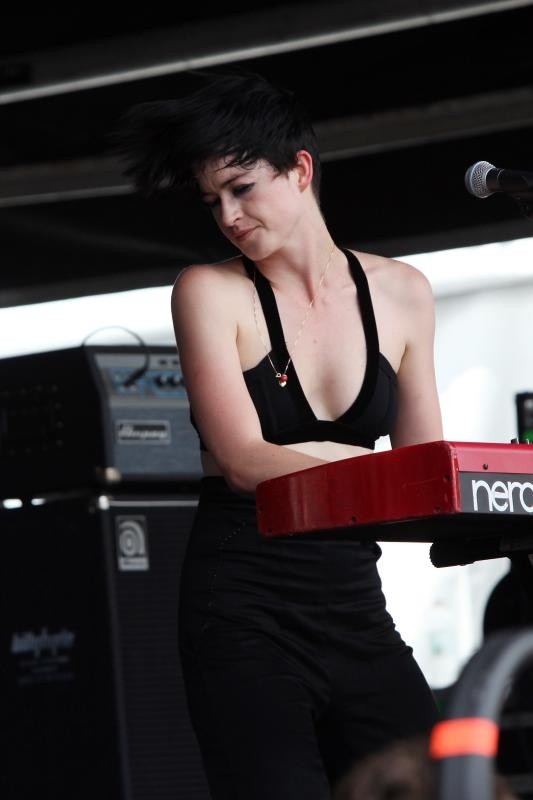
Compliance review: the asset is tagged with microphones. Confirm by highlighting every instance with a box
[464,158,533,202]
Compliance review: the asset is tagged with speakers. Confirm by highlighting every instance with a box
[1,496,212,800]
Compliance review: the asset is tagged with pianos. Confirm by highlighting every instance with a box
[254,438,533,544]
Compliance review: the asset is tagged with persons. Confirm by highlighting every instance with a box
[171,75,446,800]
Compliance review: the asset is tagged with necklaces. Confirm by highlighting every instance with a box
[254,247,336,387]
